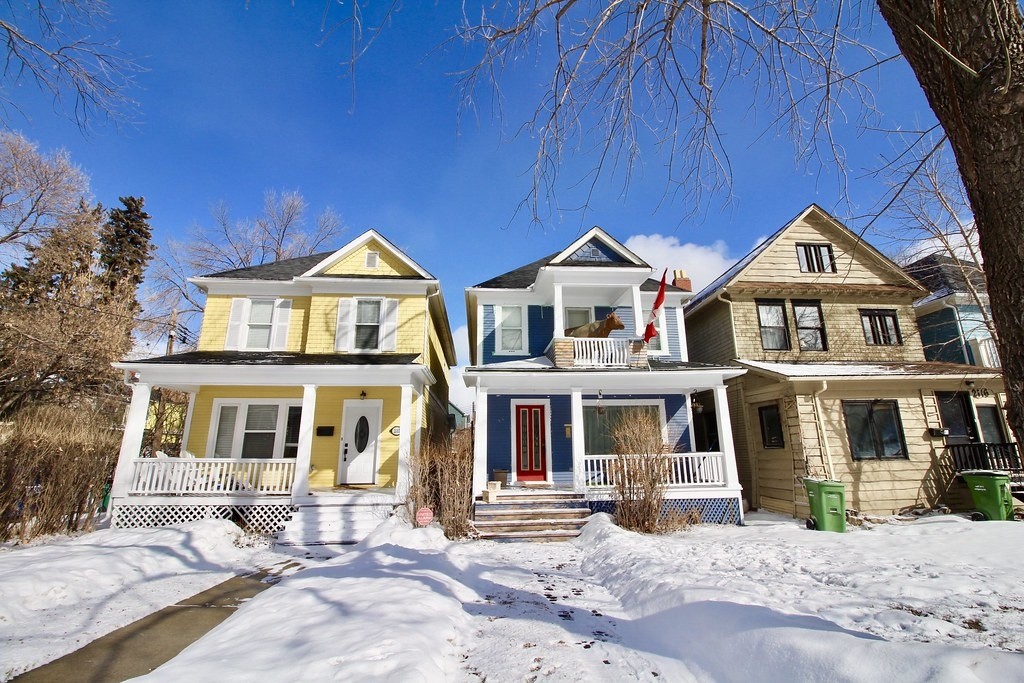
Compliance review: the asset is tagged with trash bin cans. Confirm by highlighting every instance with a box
[961,470,1015,521]
[803,477,845,533]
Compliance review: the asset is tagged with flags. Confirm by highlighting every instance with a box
[643,272,665,344]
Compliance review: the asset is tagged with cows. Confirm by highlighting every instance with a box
[564,311,626,338]
